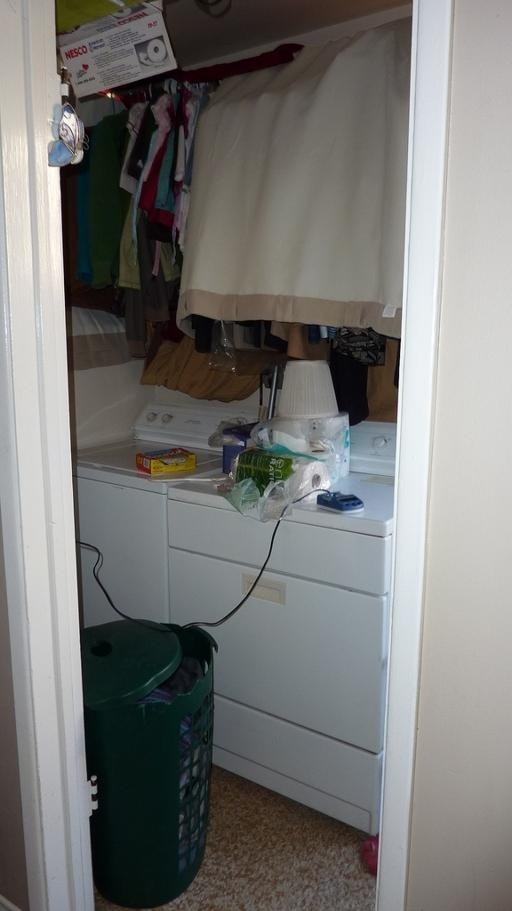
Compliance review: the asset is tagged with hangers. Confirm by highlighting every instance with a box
[120,78,215,107]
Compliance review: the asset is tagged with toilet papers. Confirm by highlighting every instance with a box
[231,449,330,501]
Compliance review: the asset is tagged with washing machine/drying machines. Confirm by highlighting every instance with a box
[76,396,259,627]
